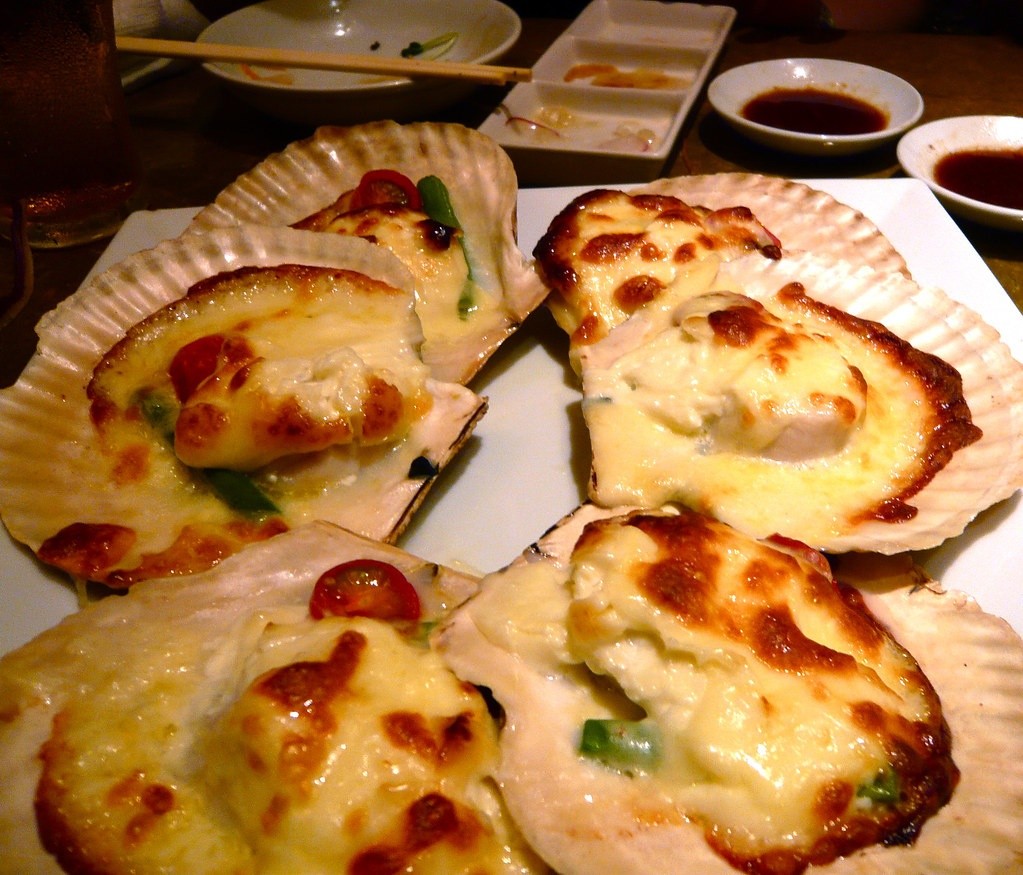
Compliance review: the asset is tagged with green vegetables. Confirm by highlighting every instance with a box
[133,172,897,803]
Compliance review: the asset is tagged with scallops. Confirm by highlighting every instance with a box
[0,121,1022,875]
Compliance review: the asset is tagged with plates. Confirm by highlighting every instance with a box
[194,0,521,103]
[896,115,1023,223]
[707,57,924,158]
[477,0,736,181]
[0,179,1023,875]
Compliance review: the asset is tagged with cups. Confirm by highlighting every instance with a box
[0,0,132,249]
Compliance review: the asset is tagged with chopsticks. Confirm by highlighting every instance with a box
[113,35,532,88]
